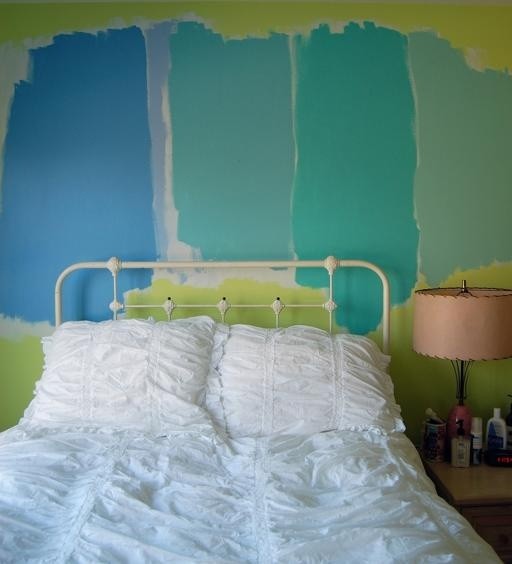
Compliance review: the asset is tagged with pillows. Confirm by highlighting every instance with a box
[204,323,402,437]
[18,314,222,446]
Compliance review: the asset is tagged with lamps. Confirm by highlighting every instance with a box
[409,284,512,432]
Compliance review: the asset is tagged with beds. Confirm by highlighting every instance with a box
[0,260,496,564]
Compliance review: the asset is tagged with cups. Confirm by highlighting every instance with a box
[419,417,447,464]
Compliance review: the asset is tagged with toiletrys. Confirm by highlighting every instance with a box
[469,417,482,464]
[451,419,470,469]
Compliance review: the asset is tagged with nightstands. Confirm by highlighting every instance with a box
[417,449,512,563]
